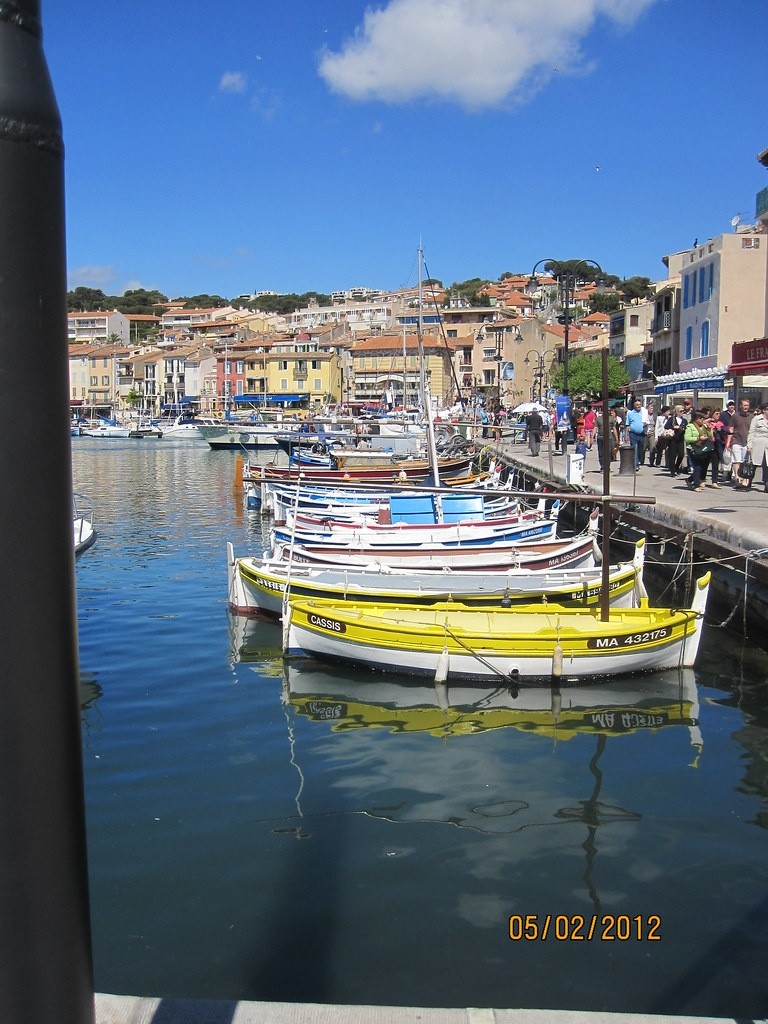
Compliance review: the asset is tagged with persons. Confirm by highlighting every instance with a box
[481,398,768,493]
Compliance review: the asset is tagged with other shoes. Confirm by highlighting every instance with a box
[700,483,707,490]
[686,479,692,488]
[677,472,681,477]
[743,483,751,490]
[711,483,723,489]
[656,465,660,468]
[670,474,677,477]
[693,488,701,491]
[636,466,639,471]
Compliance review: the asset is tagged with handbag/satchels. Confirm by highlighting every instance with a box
[658,428,675,439]
[685,440,715,461]
[737,451,755,479]
[721,451,731,472]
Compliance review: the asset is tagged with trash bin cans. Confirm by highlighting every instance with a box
[619,446,635,476]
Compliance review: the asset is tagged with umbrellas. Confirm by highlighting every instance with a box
[511,403,547,414]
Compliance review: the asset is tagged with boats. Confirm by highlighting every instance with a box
[67,228,711,686]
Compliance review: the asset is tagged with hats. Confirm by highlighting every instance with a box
[683,399,690,404]
[533,408,538,412]
[725,399,735,407]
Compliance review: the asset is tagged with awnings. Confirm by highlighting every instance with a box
[728,358,768,377]
[161,396,196,408]
[654,373,735,394]
[233,394,310,402]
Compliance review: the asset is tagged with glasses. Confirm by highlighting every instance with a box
[635,399,641,402]
[714,413,721,415]
[698,417,703,419]
[679,410,684,412]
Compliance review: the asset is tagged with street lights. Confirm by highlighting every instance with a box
[522,349,559,405]
[474,323,524,438]
[526,257,605,459]
